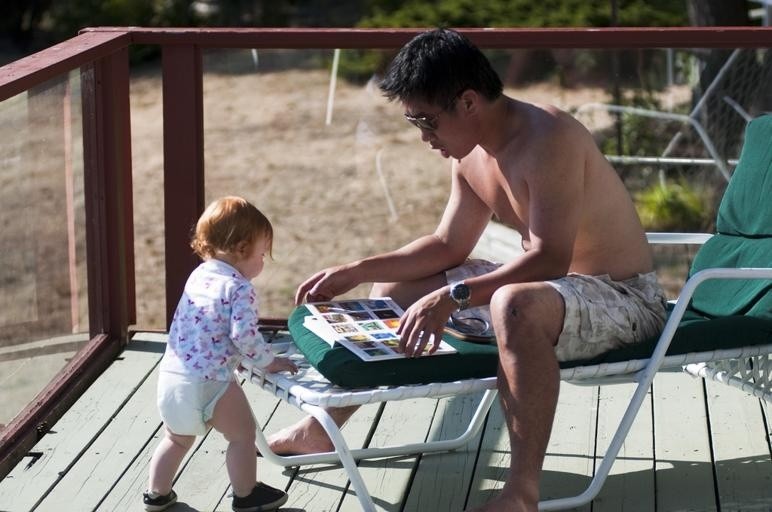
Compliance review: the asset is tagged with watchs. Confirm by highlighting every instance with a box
[450,280,472,311]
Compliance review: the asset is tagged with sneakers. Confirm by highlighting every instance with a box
[231,481,289,512]
[143,487,178,512]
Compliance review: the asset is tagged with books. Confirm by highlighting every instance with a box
[300,295,458,363]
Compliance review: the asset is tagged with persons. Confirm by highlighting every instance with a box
[241,26,672,511]
[139,192,298,509]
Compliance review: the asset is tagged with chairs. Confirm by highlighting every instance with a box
[234,115,772,512]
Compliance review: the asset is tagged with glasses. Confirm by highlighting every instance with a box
[404,101,457,130]
